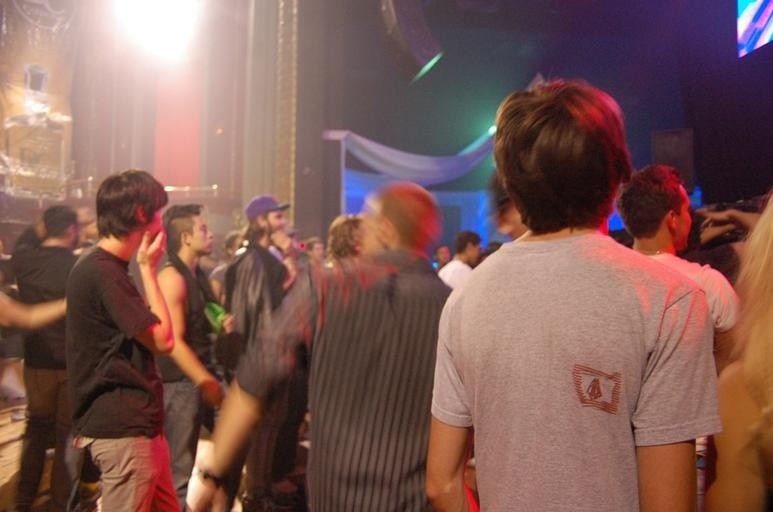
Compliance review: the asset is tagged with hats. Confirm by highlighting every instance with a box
[244,196,290,220]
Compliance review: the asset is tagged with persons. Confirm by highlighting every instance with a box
[2,83,772,511]
